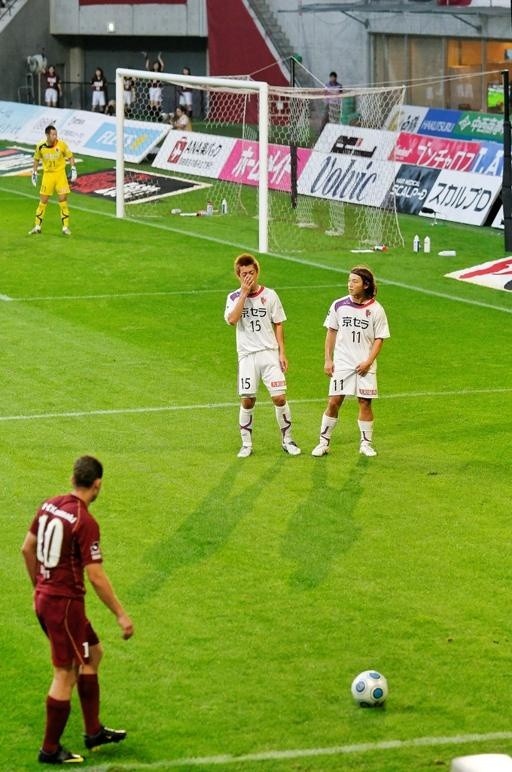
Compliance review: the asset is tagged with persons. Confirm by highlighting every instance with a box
[225,253,304,457]
[23,455,135,763]
[320,72,345,128]
[28,124,78,236]
[313,266,390,456]
[41,51,195,131]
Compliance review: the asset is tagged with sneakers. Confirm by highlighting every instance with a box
[28,227,41,234]
[63,228,71,235]
[38,746,85,764]
[282,441,301,455]
[312,444,329,457]
[238,445,252,458]
[84,727,126,750]
[360,444,377,457]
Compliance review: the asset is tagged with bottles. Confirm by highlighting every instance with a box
[424,236,431,253]
[438,250,457,256]
[221,197,228,215]
[413,234,421,252]
[206,201,214,216]
[170,208,183,215]
[369,245,387,252]
[196,211,206,217]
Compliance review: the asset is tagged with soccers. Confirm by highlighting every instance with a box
[352,670,387,706]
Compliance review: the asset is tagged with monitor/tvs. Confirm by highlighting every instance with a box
[486,84,512,111]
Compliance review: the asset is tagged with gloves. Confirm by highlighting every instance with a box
[31,171,37,186]
[70,166,77,182]
